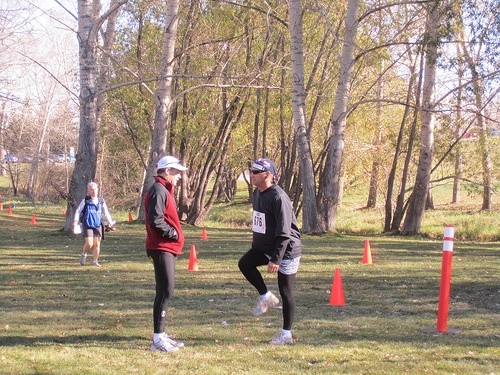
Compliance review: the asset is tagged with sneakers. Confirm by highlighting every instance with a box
[253,294,279,315]
[268,330,293,344]
[151,335,184,352]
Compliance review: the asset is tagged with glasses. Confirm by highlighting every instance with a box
[164,160,182,166]
[249,170,265,174]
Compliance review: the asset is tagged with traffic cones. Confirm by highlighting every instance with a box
[129,213,133,221]
[362,239,373,264]
[325,269,352,306]
[31,215,37,224]
[201,228,209,240]
[8,206,13,214]
[187,244,199,270]
[0,194,4,211]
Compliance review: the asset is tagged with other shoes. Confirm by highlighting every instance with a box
[80,254,87,264]
[91,261,101,267]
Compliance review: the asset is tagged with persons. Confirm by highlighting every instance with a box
[238,157,301,344]
[144,156,189,352]
[73,181,115,266]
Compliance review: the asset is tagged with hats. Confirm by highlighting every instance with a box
[252,158,276,172]
[156,156,188,172]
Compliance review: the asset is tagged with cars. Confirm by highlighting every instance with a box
[5,154,71,164]
